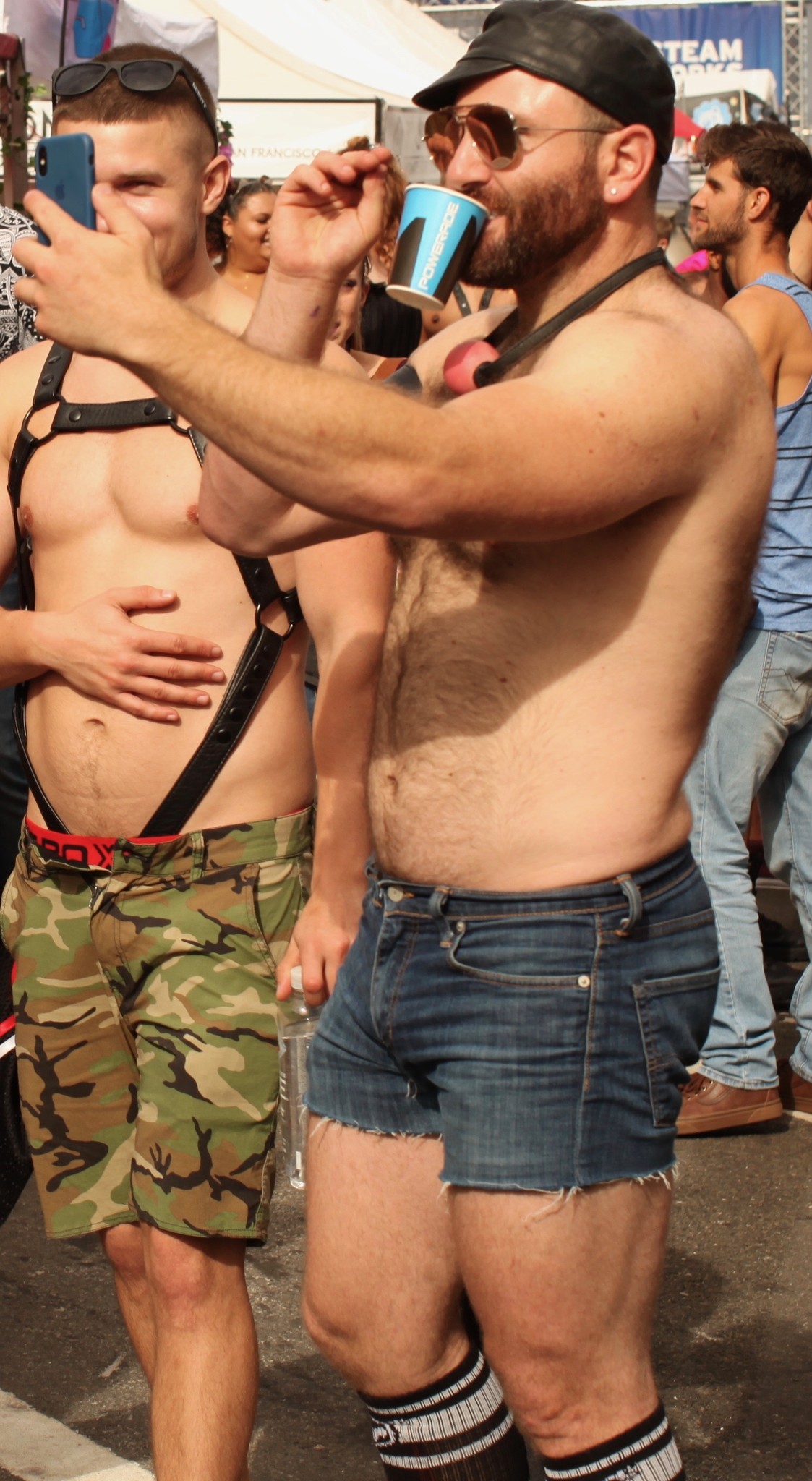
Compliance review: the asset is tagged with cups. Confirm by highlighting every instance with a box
[387,183,491,311]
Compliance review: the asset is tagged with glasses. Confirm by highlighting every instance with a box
[228,176,276,202]
[422,104,622,169]
[51,58,218,160]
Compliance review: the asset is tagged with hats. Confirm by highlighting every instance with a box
[412,0,675,164]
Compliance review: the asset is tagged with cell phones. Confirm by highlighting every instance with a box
[32,134,97,246]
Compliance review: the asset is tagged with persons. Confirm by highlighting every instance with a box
[670,120,812,1134]
[0,0,810,1481]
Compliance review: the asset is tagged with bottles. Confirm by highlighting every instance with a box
[274,971,324,1190]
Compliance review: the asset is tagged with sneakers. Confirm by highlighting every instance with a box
[779,1072,811,1120]
[662,1072,783,1133]
